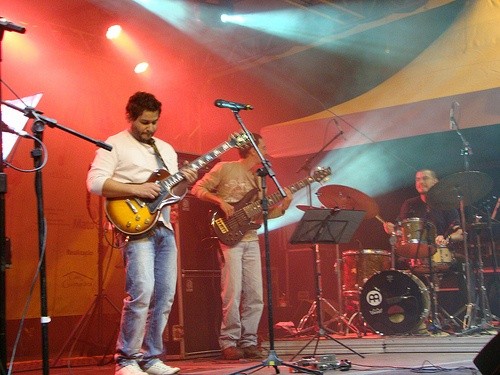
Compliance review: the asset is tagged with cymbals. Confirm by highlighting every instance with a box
[316,184,379,219]
[295,205,322,213]
[425,171,494,211]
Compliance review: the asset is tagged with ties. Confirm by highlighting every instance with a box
[145,138,169,172]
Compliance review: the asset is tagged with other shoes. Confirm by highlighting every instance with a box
[241,345,263,359]
[115,358,149,375]
[222,347,245,360]
[144,358,181,375]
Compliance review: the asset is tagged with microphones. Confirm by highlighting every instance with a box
[0,18,26,35]
[450,102,454,129]
[1,121,32,139]
[335,119,347,141]
[215,99,253,111]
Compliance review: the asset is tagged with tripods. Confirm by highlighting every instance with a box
[228,108,324,375]
[289,206,368,364]
[406,187,500,337]
[51,193,121,370]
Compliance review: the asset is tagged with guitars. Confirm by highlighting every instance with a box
[206,166,333,249]
[103,129,255,239]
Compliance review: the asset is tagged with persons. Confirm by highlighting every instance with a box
[382,169,481,333]
[86,91,198,375]
[191,131,293,360]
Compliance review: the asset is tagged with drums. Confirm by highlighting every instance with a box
[408,244,453,274]
[340,249,392,299]
[392,217,438,260]
[358,270,431,337]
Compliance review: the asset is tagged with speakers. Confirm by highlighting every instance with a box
[169,195,224,354]
[473,332,500,375]
